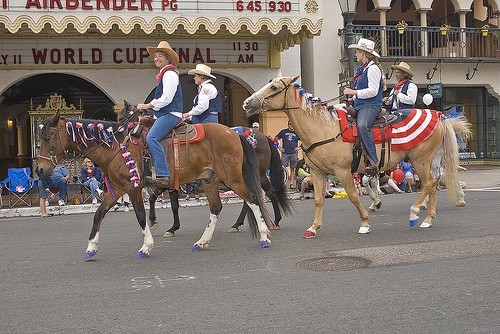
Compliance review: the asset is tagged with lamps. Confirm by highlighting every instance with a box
[440,23,448,37]
[481,25,490,36]
[396,21,408,34]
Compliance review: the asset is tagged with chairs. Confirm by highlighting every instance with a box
[0,167,164,208]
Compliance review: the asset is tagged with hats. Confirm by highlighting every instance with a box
[252,122,259,128]
[392,62,414,77]
[348,38,380,58]
[188,64,216,80]
[147,40,178,68]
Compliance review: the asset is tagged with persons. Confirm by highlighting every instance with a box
[384,62,417,110]
[182,63,222,185]
[37,119,468,216]
[344,38,388,173]
[137,40,185,186]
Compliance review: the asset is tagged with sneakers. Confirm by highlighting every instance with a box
[58,200,65,206]
[45,201,50,206]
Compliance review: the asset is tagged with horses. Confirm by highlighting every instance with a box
[332,103,476,212]
[118,100,301,237]
[242,71,466,239]
[35,109,278,259]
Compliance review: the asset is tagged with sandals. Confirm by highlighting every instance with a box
[290,184,296,189]
[398,190,405,193]
[378,191,384,195]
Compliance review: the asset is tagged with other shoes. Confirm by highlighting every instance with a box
[92,198,98,204]
[196,194,199,199]
[325,192,333,198]
[300,193,304,200]
[124,206,129,212]
[408,188,412,193]
[365,166,378,176]
[146,175,170,185]
[98,189,104,196]
[185,194,190,200]
[144,196,150,202]
[113,204,119,210]
[155,197,162,202]
